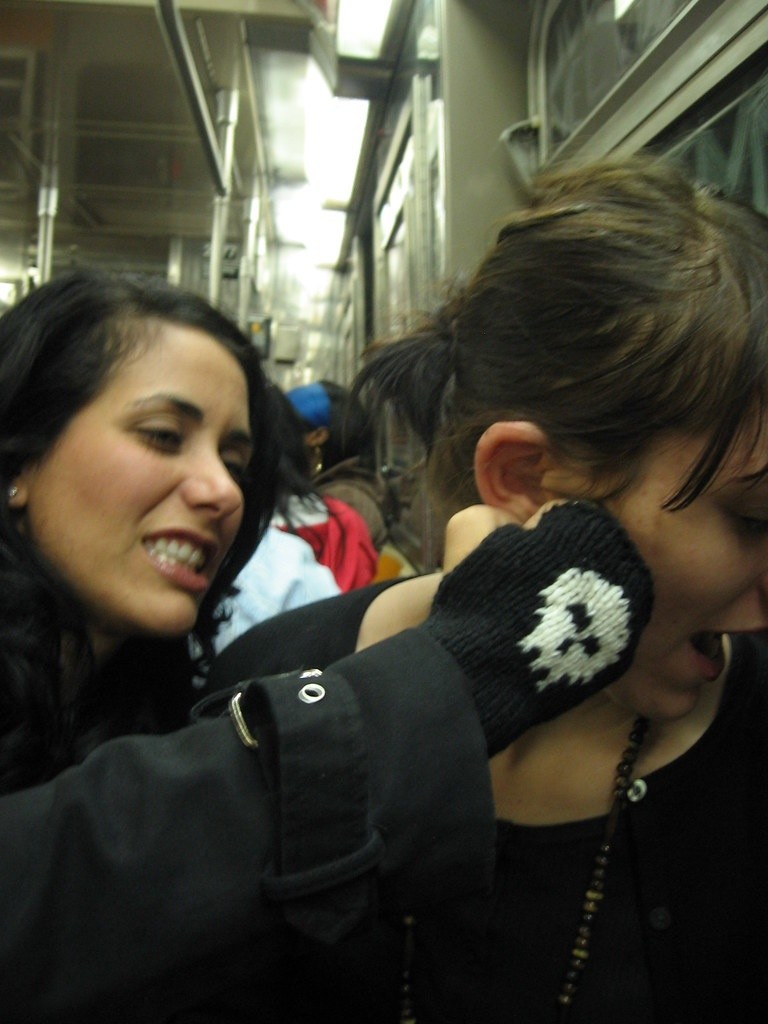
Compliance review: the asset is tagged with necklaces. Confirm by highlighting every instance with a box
[396,713,650,1024]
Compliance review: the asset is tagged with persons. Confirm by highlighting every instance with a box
[0,157,767,1023]
